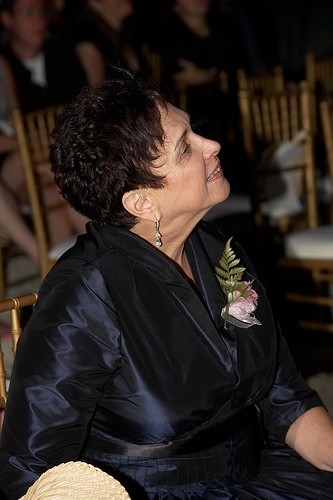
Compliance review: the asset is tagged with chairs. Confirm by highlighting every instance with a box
[0,50,333,435]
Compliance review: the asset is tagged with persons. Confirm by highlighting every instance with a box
[71,0,244,170]
[0,1,104,278]
[1,67,333,500]
